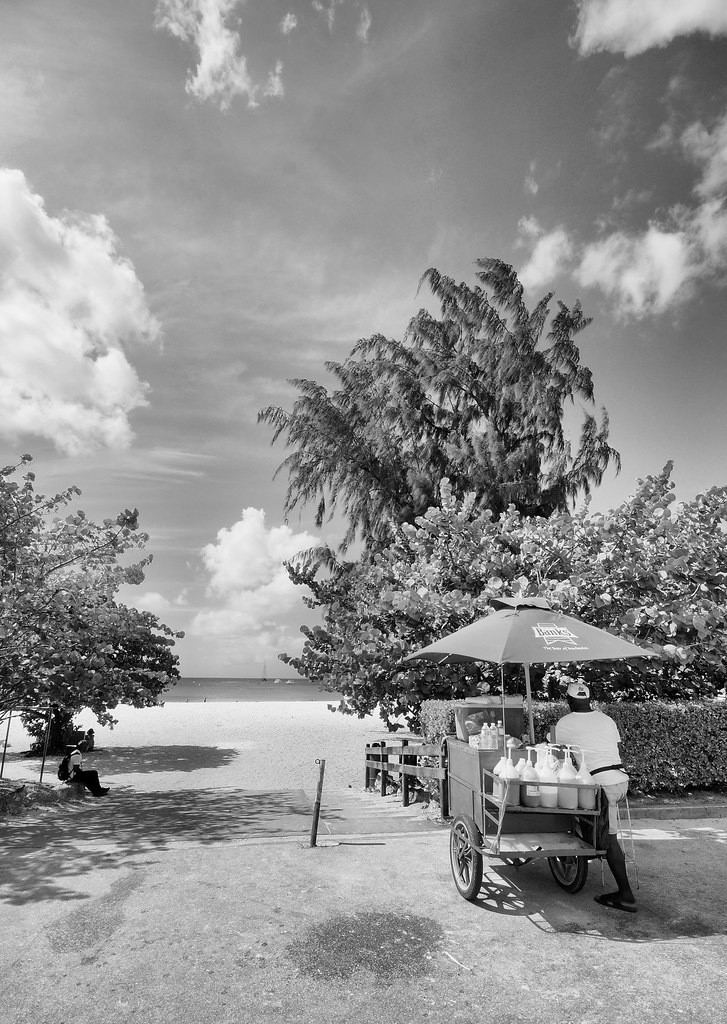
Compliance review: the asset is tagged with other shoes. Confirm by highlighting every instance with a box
[93,788,110,797]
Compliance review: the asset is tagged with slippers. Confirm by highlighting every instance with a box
[595,892,637,912]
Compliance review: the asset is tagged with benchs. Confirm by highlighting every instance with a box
[65,782,81,797]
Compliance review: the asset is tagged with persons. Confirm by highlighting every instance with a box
[66,740,110,798]
[541,682,638,913]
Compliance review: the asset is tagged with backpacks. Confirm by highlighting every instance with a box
[58,752,80,780]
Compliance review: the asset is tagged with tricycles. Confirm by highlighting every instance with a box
[439,732,603,901]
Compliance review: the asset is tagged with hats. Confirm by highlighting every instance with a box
[567,683,590,699]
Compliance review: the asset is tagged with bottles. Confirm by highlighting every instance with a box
[491,756,507,798]
[490,723,498,749]
[497,720,504,751]
[481,723,490,749]
[516,757,526,777]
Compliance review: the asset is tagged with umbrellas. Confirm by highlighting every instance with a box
[395,597,662,746]
